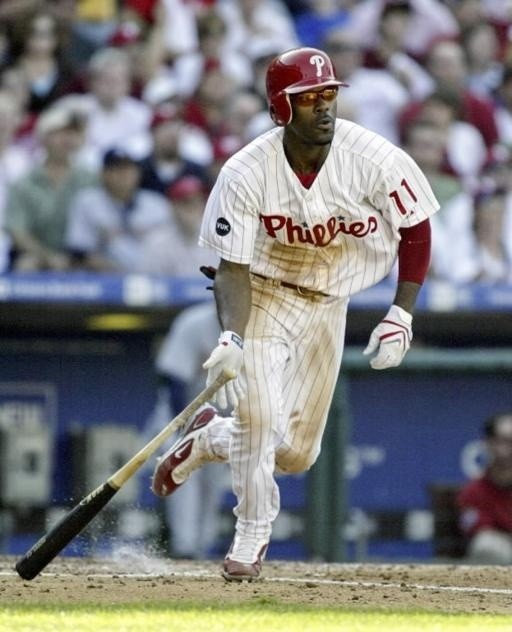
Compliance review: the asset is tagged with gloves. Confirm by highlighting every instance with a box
[362,304,414,370]
[202,330,248,410]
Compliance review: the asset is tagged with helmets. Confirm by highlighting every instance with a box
[266,47,350,127]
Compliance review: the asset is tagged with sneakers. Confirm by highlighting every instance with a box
[149,403,220,497]
[220,530,269,583]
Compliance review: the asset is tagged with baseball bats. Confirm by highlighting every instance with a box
[15,363,240,581]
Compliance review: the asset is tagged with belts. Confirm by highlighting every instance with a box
[251,272,330,297]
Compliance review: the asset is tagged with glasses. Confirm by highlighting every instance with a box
[290,88,339,106]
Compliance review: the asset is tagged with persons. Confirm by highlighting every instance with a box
[456,411,511,567]
[1,0,511,289]
[139,303,233,560]
[150,47,441,583]
